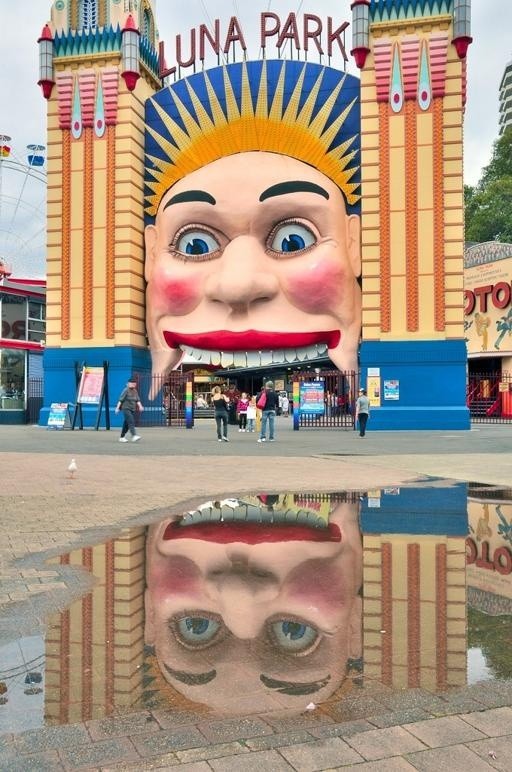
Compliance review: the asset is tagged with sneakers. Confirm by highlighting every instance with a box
[238,428,254,432]
[218,436,229,442]
[132,435,141,442]
[257,438,276,442]
[119,437,128,442]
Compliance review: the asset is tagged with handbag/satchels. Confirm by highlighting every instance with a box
[256,388,266,409]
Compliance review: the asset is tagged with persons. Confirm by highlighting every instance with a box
[0,381,18,397]
[257,495,280,512]
[196,394,207,409]
[356,388,370,438]
[324,390,344,417]
[114,379,145,443]
[211,380,289,442]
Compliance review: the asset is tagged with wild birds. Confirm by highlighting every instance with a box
[66,458,77,478]
[305,702,316,712]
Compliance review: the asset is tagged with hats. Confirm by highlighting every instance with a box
[359,388,365,391]
[128,378,137,382]
